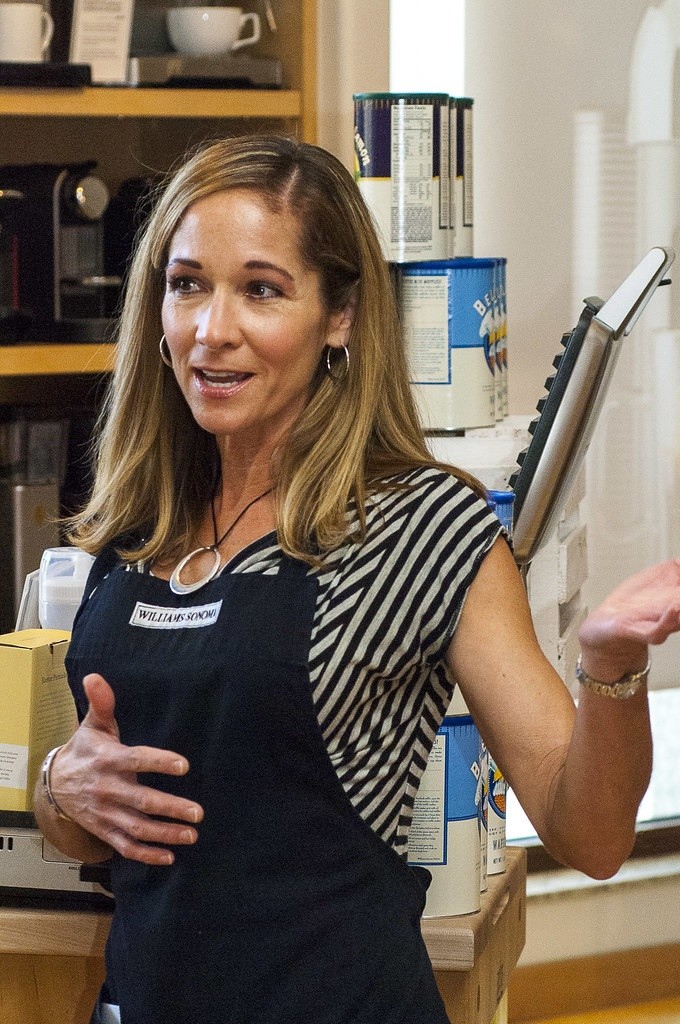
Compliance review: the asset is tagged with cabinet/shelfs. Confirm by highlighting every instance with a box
[0,0,316,378]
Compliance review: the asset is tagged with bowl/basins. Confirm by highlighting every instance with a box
[421,436,529,489]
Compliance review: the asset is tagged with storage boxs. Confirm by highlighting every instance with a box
[0,629,80,811]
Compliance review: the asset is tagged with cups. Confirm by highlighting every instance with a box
[166,6,261,55]
[0,2,54,62]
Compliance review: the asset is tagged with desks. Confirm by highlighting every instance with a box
[0,846,529,1024]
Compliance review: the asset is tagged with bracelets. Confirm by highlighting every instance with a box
[575,647,651,700]
[42,744,75,822]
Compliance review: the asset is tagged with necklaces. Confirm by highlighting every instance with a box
[169,486,276,594]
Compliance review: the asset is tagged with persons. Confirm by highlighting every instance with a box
[33,136,680,1024]
